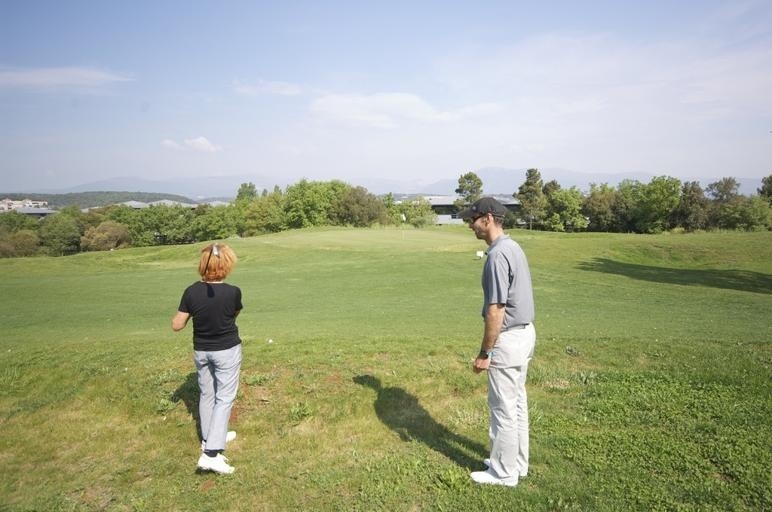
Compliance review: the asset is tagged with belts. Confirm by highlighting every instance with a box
[506,323,530,331]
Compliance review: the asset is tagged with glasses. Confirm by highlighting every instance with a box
[470,214,486,224]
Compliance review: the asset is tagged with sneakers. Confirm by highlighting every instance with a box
[470,458,527,486]
[198,452,235,474]
[201,431,236,451]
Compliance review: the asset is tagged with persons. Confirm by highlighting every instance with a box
[171,245,242,475]
[457,198,534,487]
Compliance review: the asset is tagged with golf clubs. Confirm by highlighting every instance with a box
[200,244,221,283]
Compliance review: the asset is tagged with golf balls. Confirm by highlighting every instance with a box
[268,339,273,343]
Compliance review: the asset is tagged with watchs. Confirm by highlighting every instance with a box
[478,350,492,359]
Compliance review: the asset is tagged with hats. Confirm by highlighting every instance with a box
[457,197,506,219]
